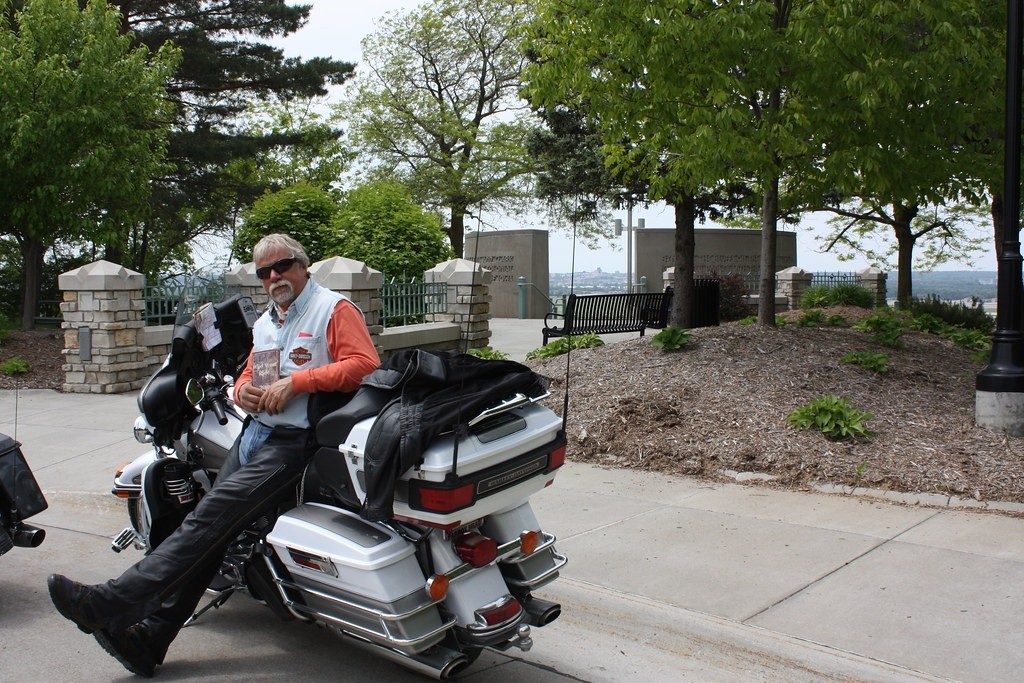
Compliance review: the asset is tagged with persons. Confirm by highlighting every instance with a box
[48,234,381,678]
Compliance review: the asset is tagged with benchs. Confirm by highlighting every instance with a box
[542,285,672,344]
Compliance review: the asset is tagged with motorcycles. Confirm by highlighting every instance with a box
[111,261,570,680]
[0,433,49,558]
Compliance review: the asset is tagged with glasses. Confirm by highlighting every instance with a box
[256,255,297,280]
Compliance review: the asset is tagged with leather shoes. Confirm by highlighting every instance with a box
[93,623,156,678]
[47,573,120,635]
